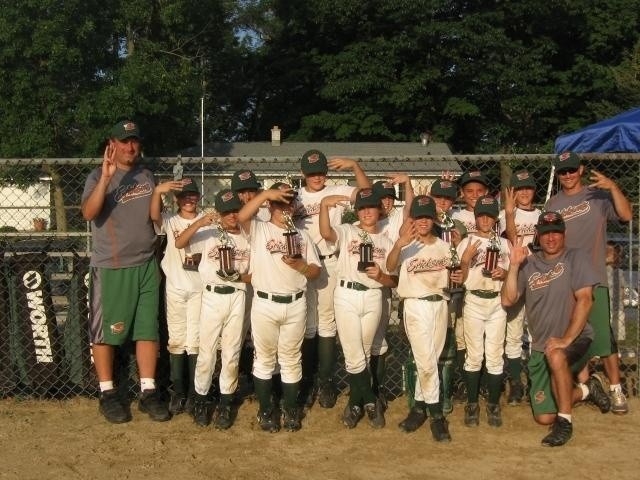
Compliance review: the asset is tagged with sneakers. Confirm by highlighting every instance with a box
[584,373,628,413]
[541,415,572,447]
[400,399,500,441]
[99,390,236,428]
[257,377,385,432]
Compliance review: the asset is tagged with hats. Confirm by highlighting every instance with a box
[412,152,580,233]
[301,150,328,174]
[112,121,140,141]
[215,170,295,211]
[174,180,199,195]
[356,181,396,208]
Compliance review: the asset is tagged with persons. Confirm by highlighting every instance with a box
[81,121,634,447]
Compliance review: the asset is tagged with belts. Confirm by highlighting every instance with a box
[471,291,498,298]
[419,294,443,301]
[319,255,333,260]
[341,280,369,290]
[257,291,303,303]
[206,285,235,294]
